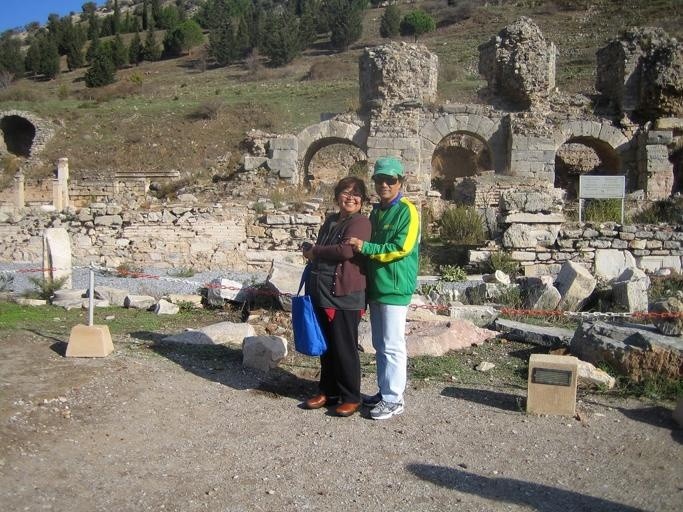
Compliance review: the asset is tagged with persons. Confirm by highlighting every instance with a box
[302,156,421,420]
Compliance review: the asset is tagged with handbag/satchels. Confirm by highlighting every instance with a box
[292,264,327,356]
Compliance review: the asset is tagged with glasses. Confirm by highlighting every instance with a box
[375,178,401,185]
[340,192,363,198]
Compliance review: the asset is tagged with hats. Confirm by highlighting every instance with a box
[371,158,404,179]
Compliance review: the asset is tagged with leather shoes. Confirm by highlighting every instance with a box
[335,402,361,417]
[305,392,340,409]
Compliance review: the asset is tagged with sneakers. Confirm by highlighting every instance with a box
[363,394,404,419]
